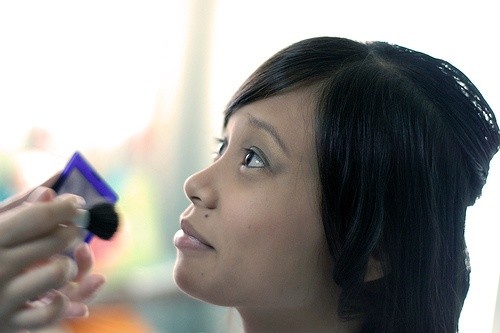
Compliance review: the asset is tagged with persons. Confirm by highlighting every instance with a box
[0,170,105,333]
[170,37,500,333]
